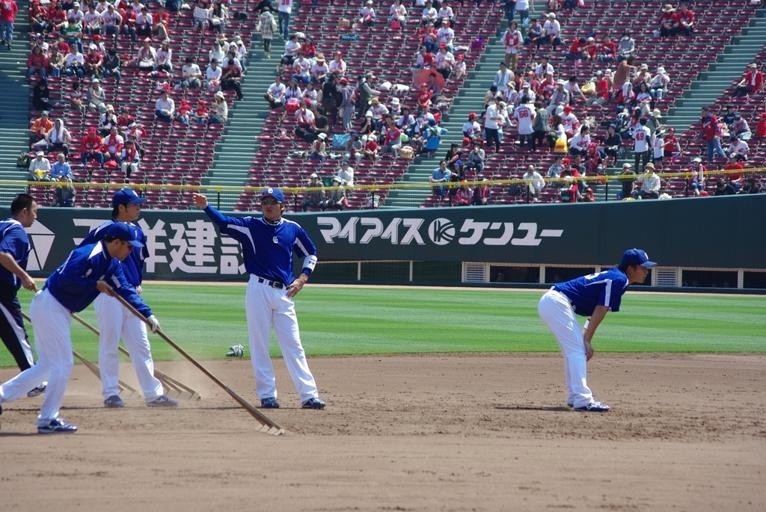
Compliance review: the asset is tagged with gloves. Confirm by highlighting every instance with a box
[147,314,161,333]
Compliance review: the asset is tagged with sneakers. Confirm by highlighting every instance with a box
[104,396,123,407]
[26,382,47,397]
[260,398,279,409]
[573,402,609,411]
[38,419,77,435]
[147,396,178,407]
[302,398,325,409]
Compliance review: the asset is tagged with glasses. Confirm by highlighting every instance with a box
[262,199,281,206]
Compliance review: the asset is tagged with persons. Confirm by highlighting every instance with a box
[0,223,161,435]
[254,1,468,212]
[75,188,179,408]
[687,52,765,196]
[192,188,326,409]
[0,194,48,397]
[1,1,19,50]
[537,249,658,413]
[428,1,696,201]
[26,1,249,207]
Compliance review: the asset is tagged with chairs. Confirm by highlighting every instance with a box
[27,0,765,212]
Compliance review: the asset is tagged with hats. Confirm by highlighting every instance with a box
[256,188,285,201]
[104,223,145,248]
[112,188,144,209]
[368,97,380,105]
[645,163,656,171]
[214,90,226,99]
[523,81,530,88]
[507,80,516,90]
[621,248,657,267]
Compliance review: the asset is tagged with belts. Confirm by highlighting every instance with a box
[259,278,284,290]
[553,286,574,307]
